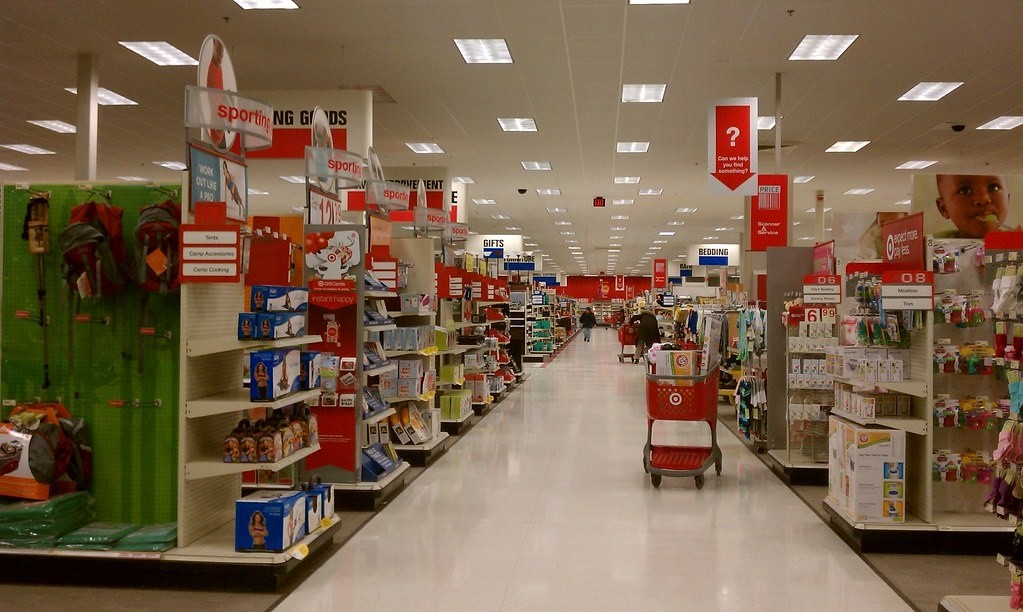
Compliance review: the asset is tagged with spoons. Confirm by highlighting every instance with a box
[977,214,1016,231]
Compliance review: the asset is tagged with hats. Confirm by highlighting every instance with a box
[586,307,591,311]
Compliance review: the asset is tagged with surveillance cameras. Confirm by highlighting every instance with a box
[952,125,965,131]
[518,189,527,194]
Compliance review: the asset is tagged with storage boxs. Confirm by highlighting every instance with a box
[238,312,258,340]
[224,413,319,462]
[369,325,435,445]
[305,492,321,535]
[234,490,306,554]
[302,483,334,519]
[700,317,722,376]
[787,318,911,524]
[656,350,697,387]
[249,349,300,401]
[258,314,306,340]
[249,284,309,313]
[300,351,321,390]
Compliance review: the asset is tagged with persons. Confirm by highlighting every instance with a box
[248,512,268,550]
[254,363,269,400]
[314,124,332,148]
[222,161,245,215]
[620,307,625,322]
[629,311,661,363]
[932,175,1020,240]
[288,510,295,545]
[241,290,292,339]
[579,307,596,342]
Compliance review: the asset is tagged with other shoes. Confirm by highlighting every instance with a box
[584,338,586,341]
[587,340,589,342]
[635,359,639,364]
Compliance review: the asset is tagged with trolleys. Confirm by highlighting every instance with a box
[643,350,723,490]
[605,313,619,330]
[617,324,638,363]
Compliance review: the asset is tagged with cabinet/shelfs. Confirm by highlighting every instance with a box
[485,313,515,391]
[525,294,580,362]
[175,168,342,591]
[306,221,410,513]
[822,233,934,554]
[590,300,673,343]
[378,308,449,466]
[768,257,842,487]
[436,349,474,436]
[480,301,512,402]
[451,319,494,415]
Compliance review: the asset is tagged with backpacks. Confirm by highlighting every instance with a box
[56,191,180,373]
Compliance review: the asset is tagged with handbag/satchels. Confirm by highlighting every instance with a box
[0,402,93,503]
